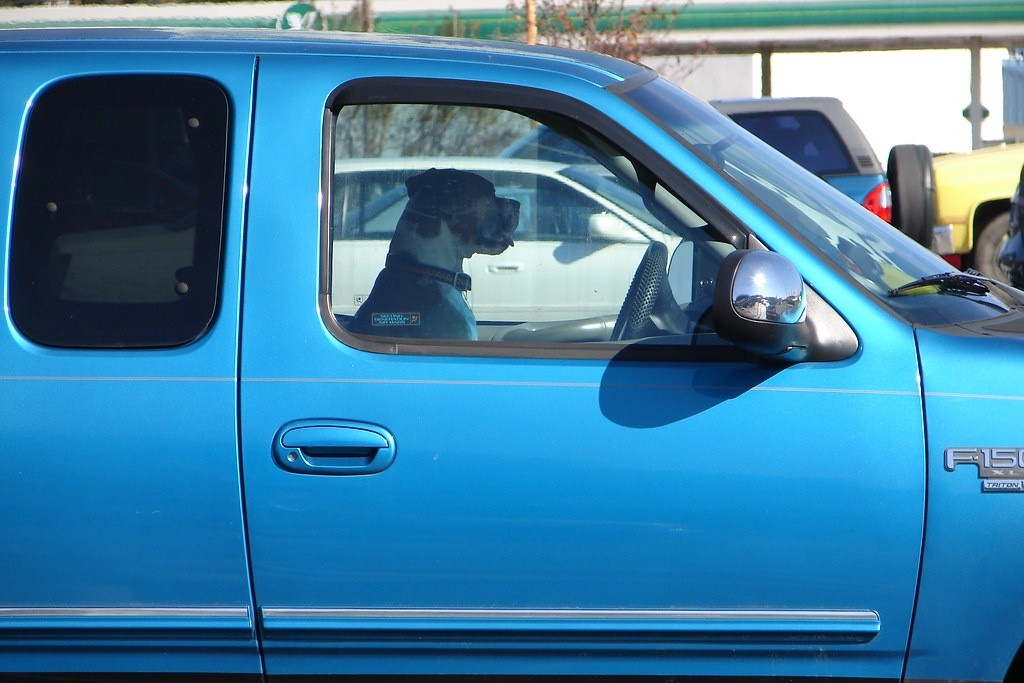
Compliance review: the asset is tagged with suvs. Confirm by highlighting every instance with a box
[888,123,1024,298]
[326,97,938,251]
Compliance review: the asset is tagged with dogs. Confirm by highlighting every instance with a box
[342,167,520,342]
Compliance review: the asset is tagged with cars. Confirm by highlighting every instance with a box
[0,1,1024,682]
[57,156,693,340]
